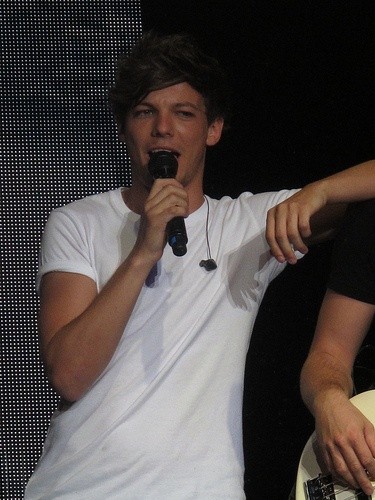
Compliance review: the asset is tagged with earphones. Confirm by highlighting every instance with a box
[199,259,217,271]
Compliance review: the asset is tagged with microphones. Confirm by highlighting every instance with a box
[147,151,188,256]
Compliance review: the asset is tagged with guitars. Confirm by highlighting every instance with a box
[295,389,375,500]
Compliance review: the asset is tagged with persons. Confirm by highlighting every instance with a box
[23,31,375,500]
[299,203,375,496]
